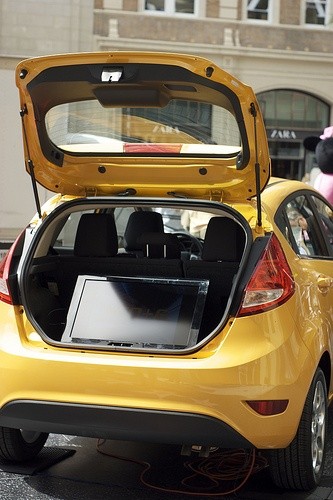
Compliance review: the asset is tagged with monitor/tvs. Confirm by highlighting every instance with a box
[62,275,210,350]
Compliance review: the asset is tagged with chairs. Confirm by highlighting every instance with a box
[122,212,166,256]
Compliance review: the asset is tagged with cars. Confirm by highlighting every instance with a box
[0,51,333,492]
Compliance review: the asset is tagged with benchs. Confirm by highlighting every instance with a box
[51,212,247,310]
